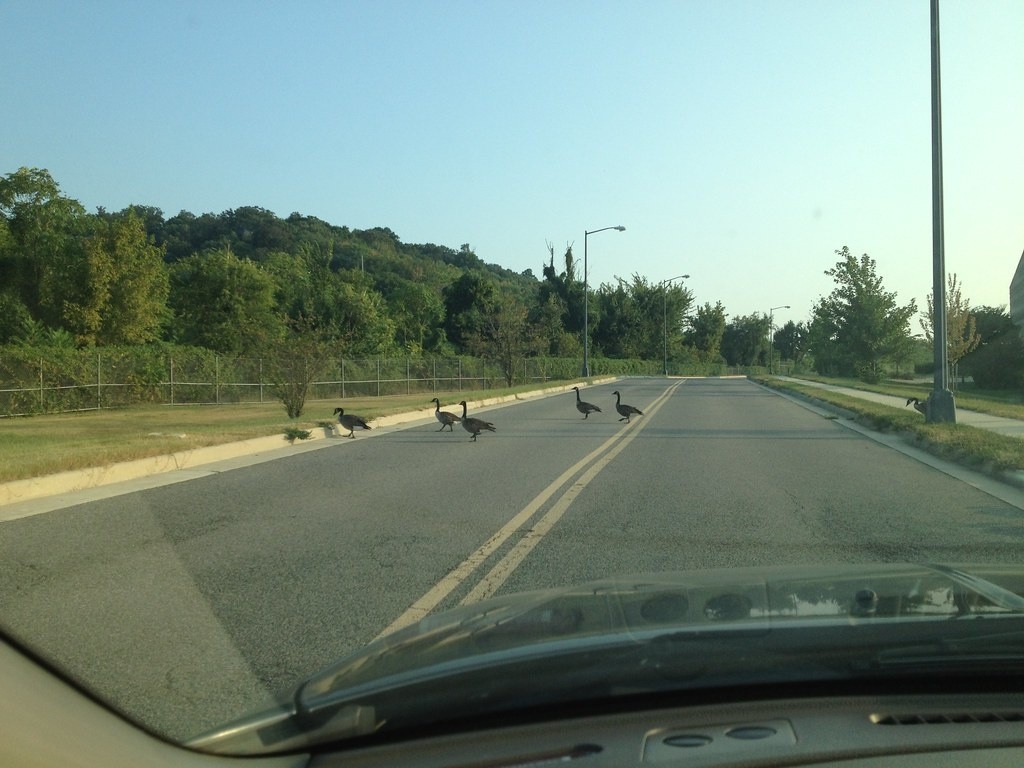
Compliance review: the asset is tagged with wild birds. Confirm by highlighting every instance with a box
[333,408,372,438]
[612,391,645,424]
[906,397,926,413]
[571,386,602,420]
[430,398,496,442]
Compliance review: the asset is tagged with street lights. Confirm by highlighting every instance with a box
[582,225,627,377]
[663,274,690,376]
[770,305,792,374]
